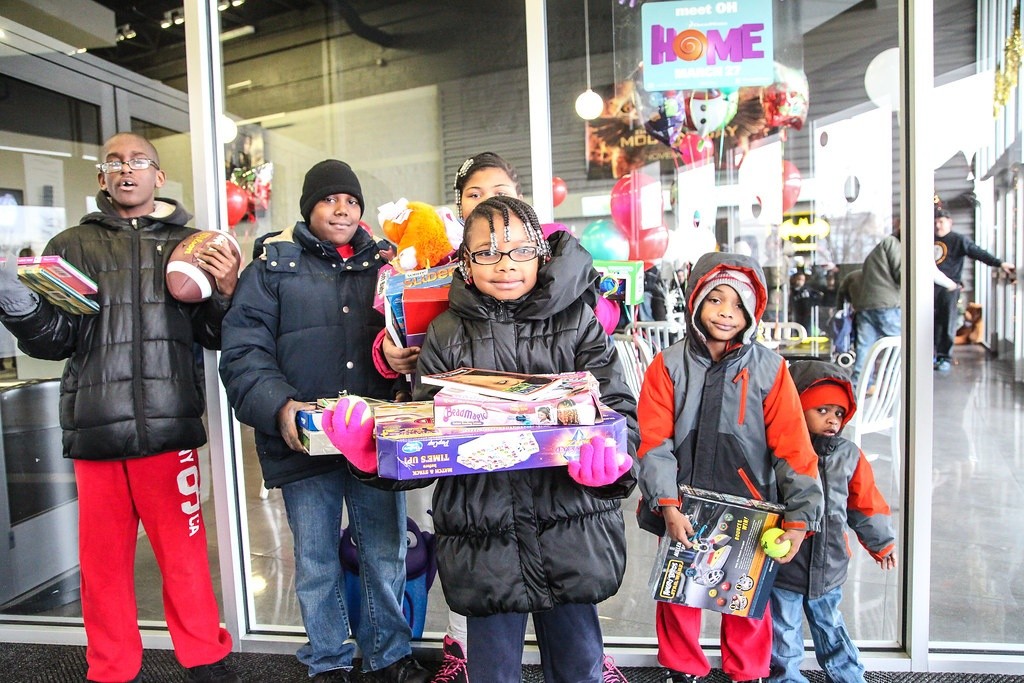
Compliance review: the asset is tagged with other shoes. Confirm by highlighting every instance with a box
[934,359,951,372]
[866,385,876,395]
[665,669,770,683]
[313,655,434,683]
[184,659,242,683]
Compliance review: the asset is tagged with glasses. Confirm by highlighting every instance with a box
[99,157,161,175]
[464,244,543,265]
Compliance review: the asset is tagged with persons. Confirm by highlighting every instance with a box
[537,407,551,425]
[219,152,1014,683]
[444,370,528,390]
[0,131,238,683]
[557,399,580,424]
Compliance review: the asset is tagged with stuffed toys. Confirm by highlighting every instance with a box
[954,302,983,344]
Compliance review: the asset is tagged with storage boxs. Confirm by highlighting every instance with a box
[431,370,604,428]
[299,425,343,456]
[374,397,629,481]
[648,483,787,621]
[297,402,326,431]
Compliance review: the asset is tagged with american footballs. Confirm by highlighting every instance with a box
[165,228,242,304]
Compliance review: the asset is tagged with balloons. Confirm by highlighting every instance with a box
[633,55,811,214]
[578,217,629,262]
[552,174,567,209]
[609,173,664,240]
[228,179,247,227]
[630,215,670,271]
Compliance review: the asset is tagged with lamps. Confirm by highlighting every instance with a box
[160,11,173,29]
[116,33,125,42]
[174,9,185,25]
[122,25,137,39]
[231,0,245,7]
[217,0,230,12]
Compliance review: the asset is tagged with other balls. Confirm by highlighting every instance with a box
[335,395,373,427]
[760,528,792,559]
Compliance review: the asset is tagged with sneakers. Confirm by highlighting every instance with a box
[432,636,468,683]
[602,654,628,683]
[84,669,141,683]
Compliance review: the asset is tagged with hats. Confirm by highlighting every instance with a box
[934,208,949,217]
[300,159,365,224]
[800,384,850,415]
[692,271,758,345]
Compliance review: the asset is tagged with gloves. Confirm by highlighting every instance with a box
[321,399,377,473]
[0,240,39,316]
[568,436,633,488]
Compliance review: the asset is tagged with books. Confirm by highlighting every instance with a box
[420,366,564,402]
[0,256,100,317]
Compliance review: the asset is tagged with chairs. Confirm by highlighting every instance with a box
[762,321,808,340]
[843,336,901,496]
[611,333,653,406]
[625,321,683,358]
